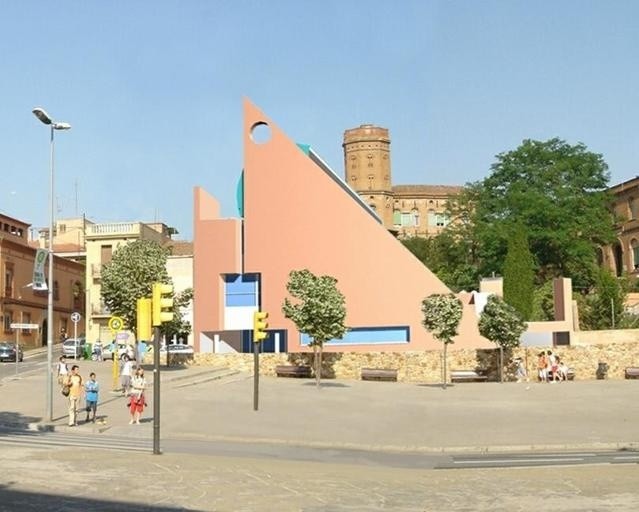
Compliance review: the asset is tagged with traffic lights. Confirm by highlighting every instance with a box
[150,282,175,327]
[253,312,272,343]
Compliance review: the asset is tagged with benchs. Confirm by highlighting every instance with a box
[538,368,574,379]
[450,370,488,381]
[276,366,311,378]
[362,368,397,381]
[625,367,639,379]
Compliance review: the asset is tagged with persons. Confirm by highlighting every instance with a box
[514,357,529,383]
[118,355,133,397]
[57,357,69,385]
[94,339,103,362]
[109,341,114,361]
[84,373,99,423]
[537,351,568,384]
[63,365,83,426]
[129,367,146,424]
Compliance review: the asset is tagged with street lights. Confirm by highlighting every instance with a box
[33,106,71,421]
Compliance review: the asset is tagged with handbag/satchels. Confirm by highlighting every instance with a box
[62,375,72,397]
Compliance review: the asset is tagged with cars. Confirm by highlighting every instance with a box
[0,341,24,363]
[64,337,86,358]
[159,344,194,362]
[90,342,135,362]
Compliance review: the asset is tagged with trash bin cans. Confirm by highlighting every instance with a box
[553,332,569,346]
[84,343,92,360]
